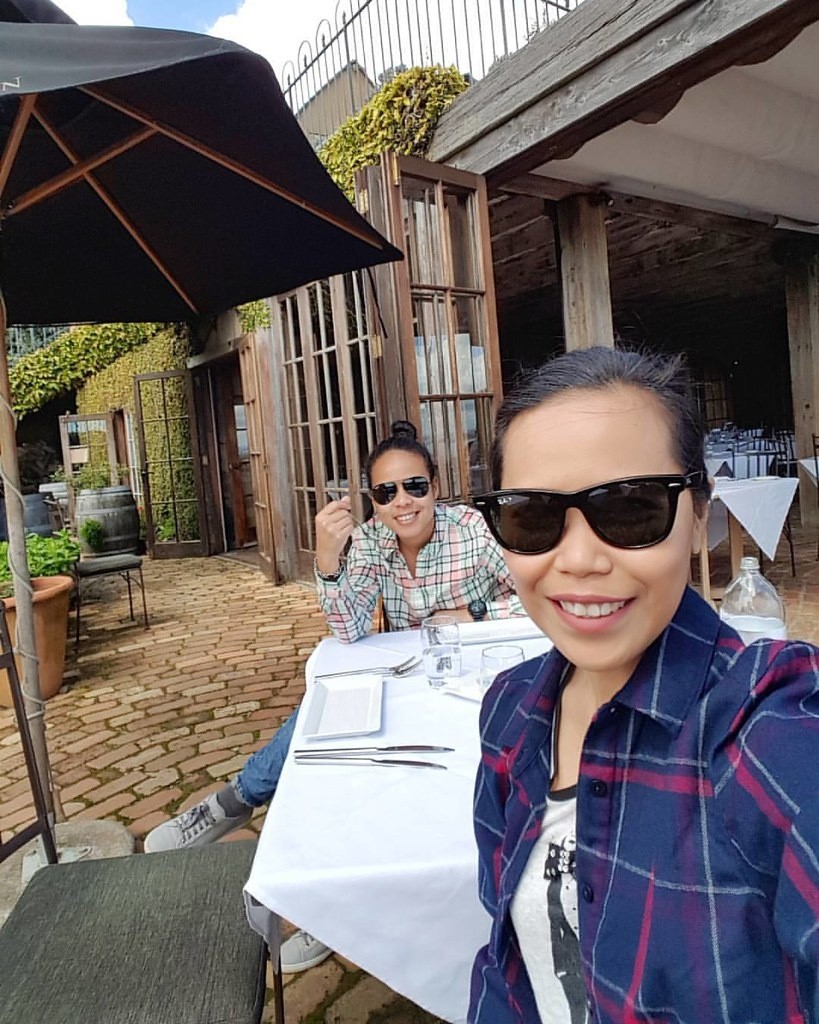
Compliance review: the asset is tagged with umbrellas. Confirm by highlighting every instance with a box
[1,0,403,864]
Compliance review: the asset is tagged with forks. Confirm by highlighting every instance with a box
[313,658,423,679]
[314,655,416,678]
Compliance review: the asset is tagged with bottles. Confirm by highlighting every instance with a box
[718,557,788,648]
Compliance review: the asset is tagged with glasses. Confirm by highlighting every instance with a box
[471,469,710,556]
[371,475,432,505]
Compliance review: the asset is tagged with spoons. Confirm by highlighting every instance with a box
[335,497,380,541]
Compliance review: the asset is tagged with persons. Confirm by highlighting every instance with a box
[467,344,819,1024]
[144,421,529,972]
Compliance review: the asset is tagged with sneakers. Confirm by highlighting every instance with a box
[280,930,333,973]
[143,793,253,853]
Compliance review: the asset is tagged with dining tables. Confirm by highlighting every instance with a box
[242,617,553,1023]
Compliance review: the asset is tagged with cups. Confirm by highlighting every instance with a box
[479,645,526,695]
[420,615,462,690]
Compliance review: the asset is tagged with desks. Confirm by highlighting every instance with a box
[798,457,819,488]
[704,452,773,477]
[690,477,800,604]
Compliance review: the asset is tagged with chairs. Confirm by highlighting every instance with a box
[43,499,150,642]
[0,601,271,1024]
[703,412,819,579]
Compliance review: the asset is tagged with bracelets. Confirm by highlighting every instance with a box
[314,558,345,579]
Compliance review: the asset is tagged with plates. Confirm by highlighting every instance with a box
[299,672,384,740]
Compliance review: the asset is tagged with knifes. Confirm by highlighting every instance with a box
[294,756,447,771]
[294,745,455,756]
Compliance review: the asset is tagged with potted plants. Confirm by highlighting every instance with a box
[0,576,75,708]
[70,463,139,559]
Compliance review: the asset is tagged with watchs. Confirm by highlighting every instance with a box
[468,600,487,622]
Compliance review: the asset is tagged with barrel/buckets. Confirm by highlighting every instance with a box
[74,485,139,559]
[0,491,56,542]
